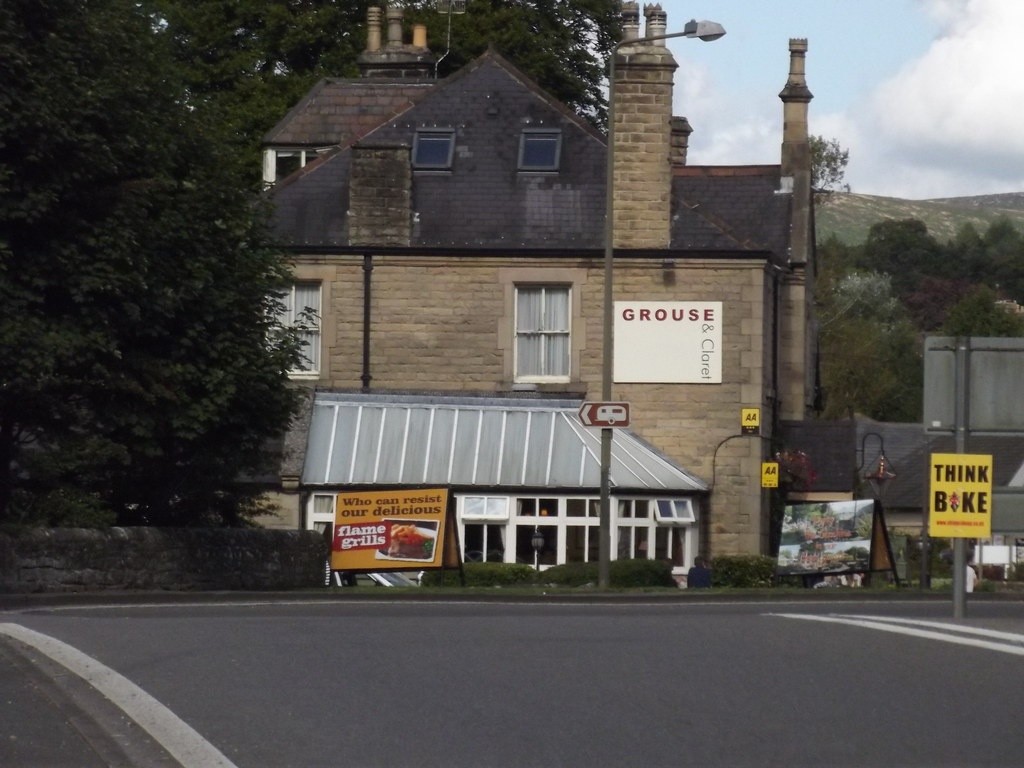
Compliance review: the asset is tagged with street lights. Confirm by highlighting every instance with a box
[594,19,728,591]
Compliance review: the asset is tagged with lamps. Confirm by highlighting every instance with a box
[857,432,896,499]
[532,529,544,550]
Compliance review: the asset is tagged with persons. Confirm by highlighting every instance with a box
[686,555,711,587]
[967,562,978,593]
